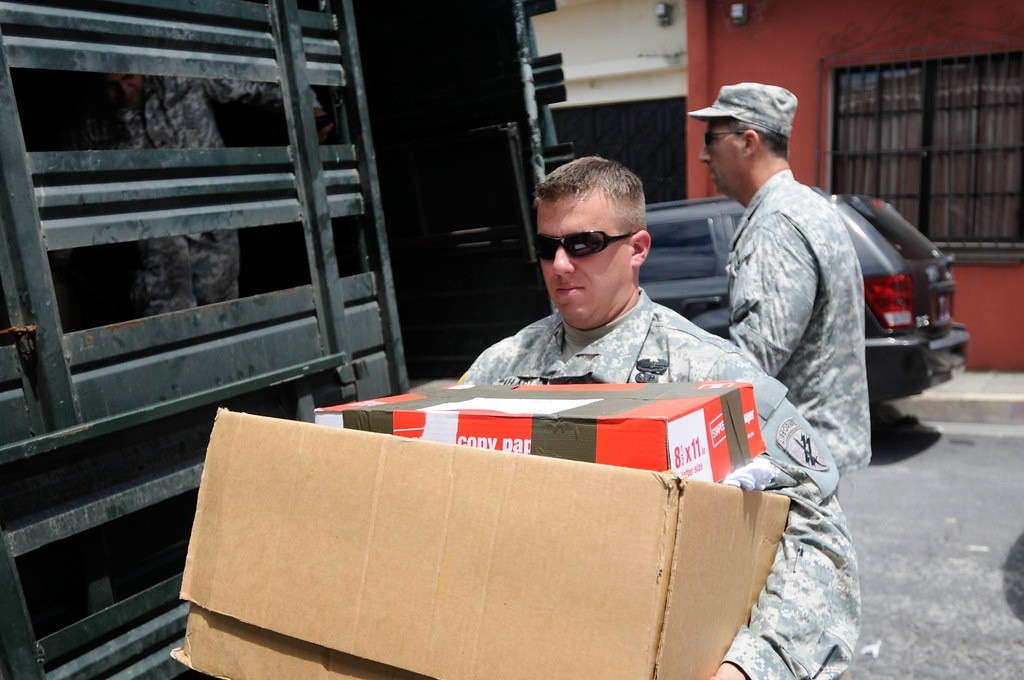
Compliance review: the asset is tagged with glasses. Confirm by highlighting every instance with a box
[534,230,637,261]
[705,130,743,144]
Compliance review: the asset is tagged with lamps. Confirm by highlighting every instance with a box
[730,3,748,25]
[655,3,672,26]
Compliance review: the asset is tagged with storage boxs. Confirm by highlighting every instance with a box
[313,381,768,484]
[165,409,792,680]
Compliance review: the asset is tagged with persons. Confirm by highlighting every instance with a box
[688,82,871,472]
[43,59,332,318]
[460,157,862,680]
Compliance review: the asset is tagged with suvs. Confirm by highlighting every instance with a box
[637,186,970,426]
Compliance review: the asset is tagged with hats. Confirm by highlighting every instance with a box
[687,82,798,138]
